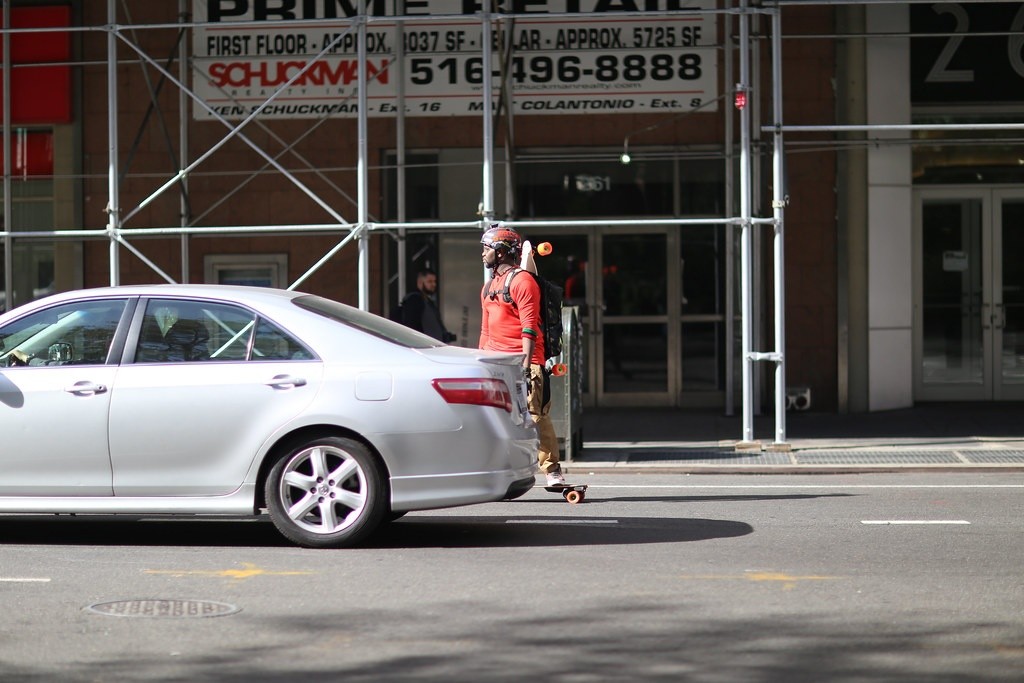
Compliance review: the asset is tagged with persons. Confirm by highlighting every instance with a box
[479,228,565,487]
[11,309,122,367]
[402,270,448,343]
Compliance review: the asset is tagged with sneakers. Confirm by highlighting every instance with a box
[546,463,565,486]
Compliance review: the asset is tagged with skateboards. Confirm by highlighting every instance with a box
[519,240,567,423]
[509,484,588,504]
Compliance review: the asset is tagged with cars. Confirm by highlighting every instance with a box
[0,282,539,550]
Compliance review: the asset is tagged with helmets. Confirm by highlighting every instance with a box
[480,228,522,260]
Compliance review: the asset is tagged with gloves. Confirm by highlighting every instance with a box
[523,367,532,396]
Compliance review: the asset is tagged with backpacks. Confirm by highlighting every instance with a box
[483,268,563,361]
